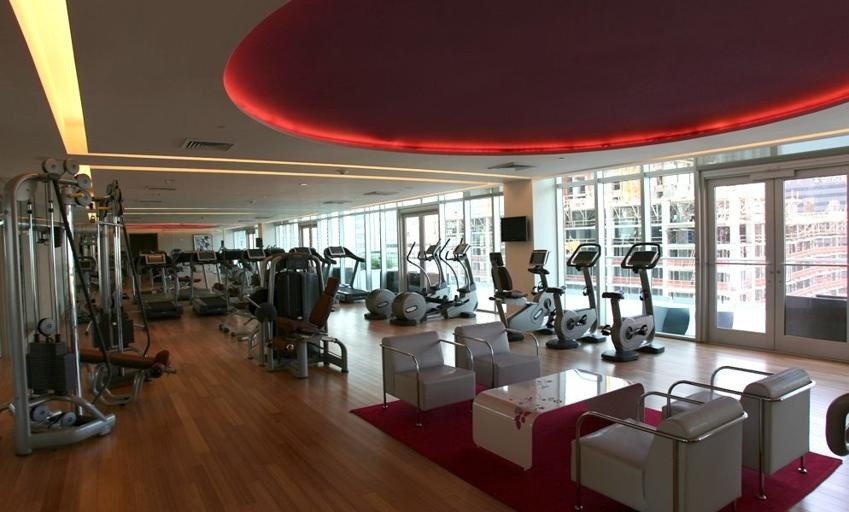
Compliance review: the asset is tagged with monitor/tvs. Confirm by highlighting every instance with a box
[196,250,217,261]
[247,248,266,259]
[425,245,436,254]
[500,216,528,243]
[452,244,470,255]
[627,250,657,266]
[129,232,158,257]
[329,246,345,256]
[143,253,166,266]
[571,250,597,264]
[294,247,312,254]
[79,259,92,271]
[528,249,550,265]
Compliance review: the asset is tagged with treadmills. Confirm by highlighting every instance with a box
[325,248,370,305]
[189,251,228,314]
[141,252,183,321]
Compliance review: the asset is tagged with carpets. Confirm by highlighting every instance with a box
[349,382,841,512]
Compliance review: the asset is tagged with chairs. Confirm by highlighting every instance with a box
[661,366,814,498]
[454,321,541,387]
[380,331,475,426]
[570,393,749,512]
